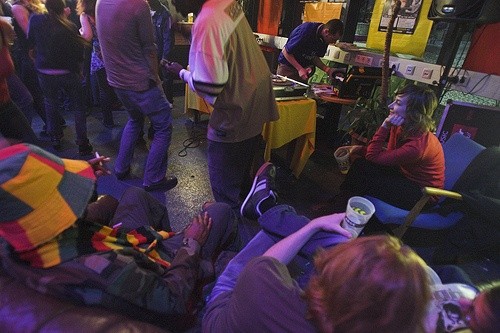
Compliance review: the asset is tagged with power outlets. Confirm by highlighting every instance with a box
[391,62,400,71]
[454,75,470,87]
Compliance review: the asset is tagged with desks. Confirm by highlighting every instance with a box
[312,84,357,137]
[184,83,317,179]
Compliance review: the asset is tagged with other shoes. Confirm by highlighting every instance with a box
[43,118,65,130]
[79,144,93,156]
[203,201,215,212]
[306,200,345,216]
[143,176,177,191]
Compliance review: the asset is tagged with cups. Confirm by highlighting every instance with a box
[334,148,351,175]
[341,196,375,238]
[0,16,14,45]
[188,13,194,23]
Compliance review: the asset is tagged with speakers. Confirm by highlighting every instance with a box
[427,0,500,26]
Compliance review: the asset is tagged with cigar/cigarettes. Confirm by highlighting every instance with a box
[94,151,104,167]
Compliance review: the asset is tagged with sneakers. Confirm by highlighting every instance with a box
[240,162,279,219]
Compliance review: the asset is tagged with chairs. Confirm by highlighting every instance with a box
[364,131,488,240]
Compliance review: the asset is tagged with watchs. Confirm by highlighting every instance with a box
[182,237,190,247]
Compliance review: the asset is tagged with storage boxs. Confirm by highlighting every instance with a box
[327,66,392,99]
[436,99,500,148]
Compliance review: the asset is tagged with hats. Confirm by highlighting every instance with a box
[0,143,94,251]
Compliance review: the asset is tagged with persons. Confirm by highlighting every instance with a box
[201,161,442,333]
[0,128,177,225]
[0,144,244,333]
[313,86,446,221]
[277,19,345,134]
[91,0,176,192]
[0,0,175,169]
[166,0,280,216]
[421,254,500,332]
